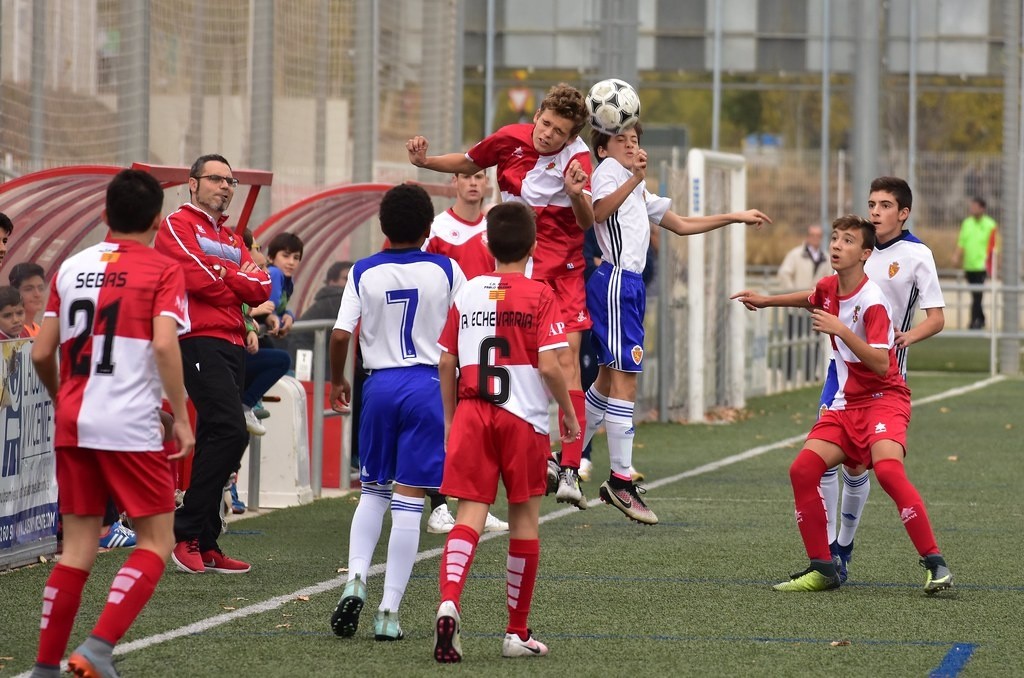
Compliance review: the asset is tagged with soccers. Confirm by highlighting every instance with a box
[584,78,641,137]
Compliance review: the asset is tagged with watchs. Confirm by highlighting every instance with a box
[211,264,222,278]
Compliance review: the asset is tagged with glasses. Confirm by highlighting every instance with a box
[196,175,240,187]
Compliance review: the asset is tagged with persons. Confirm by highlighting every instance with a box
[777,222,833,388]
[25,168,195,678]
[0,84,774,663]
[731,215,954,595]
[949,197,1002,333]
[818,177,947,583]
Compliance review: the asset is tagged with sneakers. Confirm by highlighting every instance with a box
[555,468,581,504]
[330,572,367,637]
[243,409,265,435]
[375,609,404,641]
[427,504,456,534]
[773,570,839,592]
[573,487,587,510]
[171,539,204,573]
[434,600,462,663]
[546,452,561,496]
[918,554,953,594]
[65,644,120,678]
[98,521,137,552]
[837,566,847,582]
[789,556,842,579]
[253,401,271,419]
[599,479,658,526]
[578,458,592,481]
[502,628,549,657]
[201,548,251,573]
[632,473,644,483]
[484,511,509,532]
